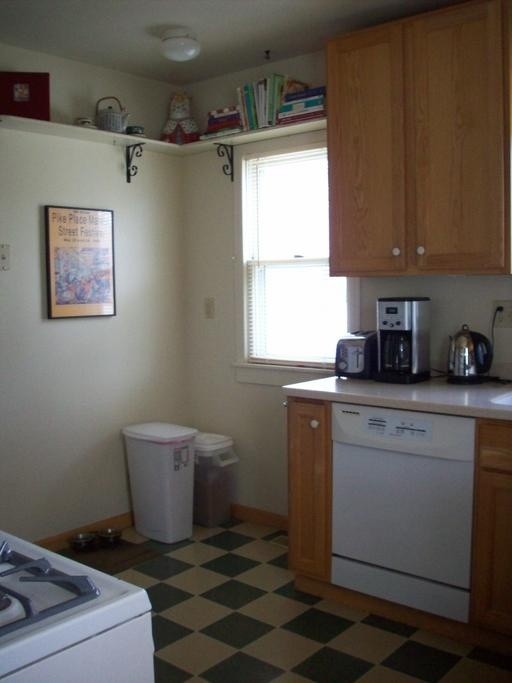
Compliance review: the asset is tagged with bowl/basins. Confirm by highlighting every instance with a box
[68,531,96,551]
[96,526,122,548]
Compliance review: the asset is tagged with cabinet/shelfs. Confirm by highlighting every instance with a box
[286,396,333,597]
[468,417,511,670]
[324,0,512,276]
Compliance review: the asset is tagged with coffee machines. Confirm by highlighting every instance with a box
[373,293,432,382]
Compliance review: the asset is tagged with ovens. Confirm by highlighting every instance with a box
[329,402,476,629]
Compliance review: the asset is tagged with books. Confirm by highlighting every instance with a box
[200,72,326,140]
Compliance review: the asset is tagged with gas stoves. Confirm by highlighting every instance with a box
[0,526,154,683]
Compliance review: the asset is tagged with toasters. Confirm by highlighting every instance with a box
[332,329,376,379]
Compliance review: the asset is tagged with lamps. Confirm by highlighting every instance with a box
[161,27,200,62]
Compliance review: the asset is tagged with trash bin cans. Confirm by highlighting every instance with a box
[121,422,199,544]
[196,432,240,528]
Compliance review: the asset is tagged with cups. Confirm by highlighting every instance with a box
[127,125,145,136]
[77,118,93,128]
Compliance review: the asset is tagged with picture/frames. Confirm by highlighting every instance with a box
[44,205,117,319]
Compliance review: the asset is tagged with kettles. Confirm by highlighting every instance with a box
[445,322,493,383]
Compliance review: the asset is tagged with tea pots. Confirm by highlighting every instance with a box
[95,95,132,133]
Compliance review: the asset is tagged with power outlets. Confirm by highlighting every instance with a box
[493,300,512,328]
